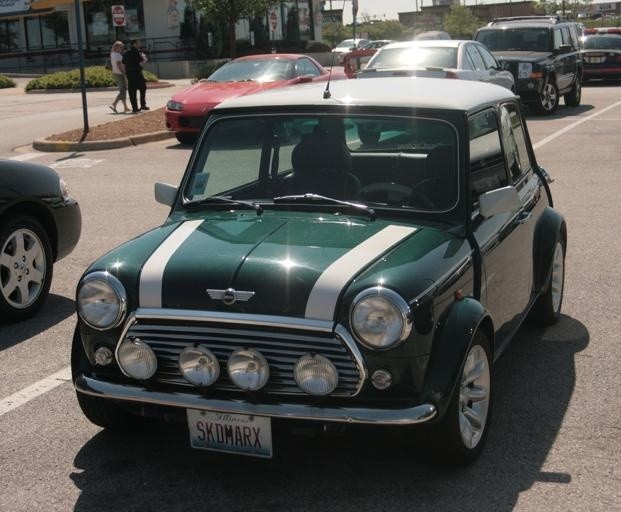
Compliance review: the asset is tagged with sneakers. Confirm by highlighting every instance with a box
[109,104,151,115]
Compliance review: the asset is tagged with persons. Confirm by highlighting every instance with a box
[122,38,150,113]
[109,40,132,114]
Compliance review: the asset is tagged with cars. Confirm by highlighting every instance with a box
[0,159,81,321]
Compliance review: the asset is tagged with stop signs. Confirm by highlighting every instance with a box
[112,5,126,27]
[270,12,278,29]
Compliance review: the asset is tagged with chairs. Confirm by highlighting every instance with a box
[281,132,362,202]
[410,146,458,208]
